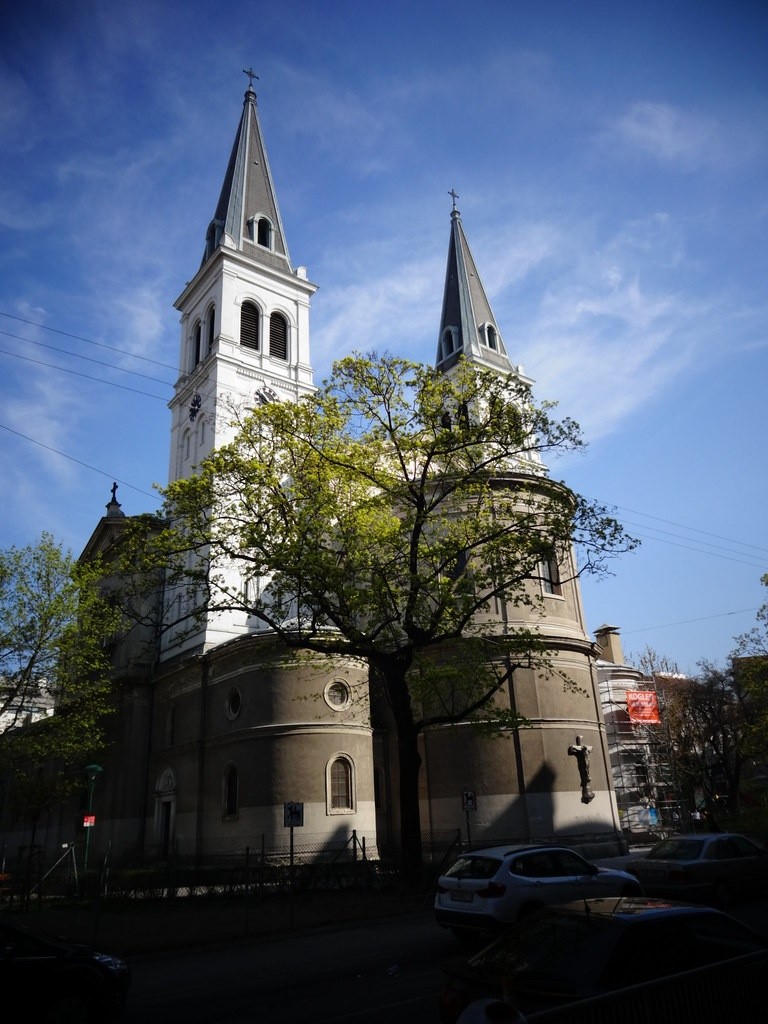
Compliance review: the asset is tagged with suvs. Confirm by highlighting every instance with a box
[432,841,646,937]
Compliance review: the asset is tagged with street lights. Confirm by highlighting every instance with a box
[79,762,105,871]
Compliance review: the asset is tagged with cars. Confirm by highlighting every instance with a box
[625,831,767,903]
[437,895,767,1023]
